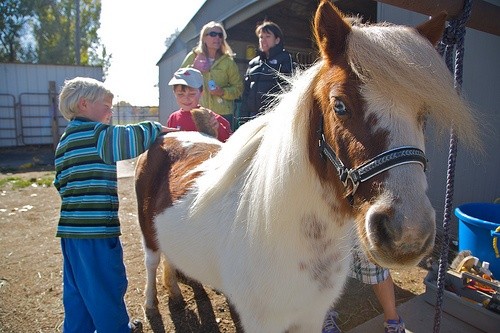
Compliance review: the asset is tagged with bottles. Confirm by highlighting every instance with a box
[479,261,493,279]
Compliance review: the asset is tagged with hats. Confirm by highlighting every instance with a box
[168,67,204,88]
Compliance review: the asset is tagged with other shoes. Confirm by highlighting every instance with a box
[128,320,143,333]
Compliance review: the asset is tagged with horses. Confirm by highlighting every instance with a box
[134,0,481,333]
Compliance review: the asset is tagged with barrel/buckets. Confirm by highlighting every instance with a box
[453,202,500,283]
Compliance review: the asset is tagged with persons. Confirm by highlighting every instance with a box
[52,76,180,333]
[321,238,406,333]
[166,68,231,143]
[180,21,245,133]
[232,22,292,133]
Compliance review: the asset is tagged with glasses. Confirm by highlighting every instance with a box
[205,31,223,38]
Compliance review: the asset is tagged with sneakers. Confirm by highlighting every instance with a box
[321,309,343,333]
[381,315,407,333]
